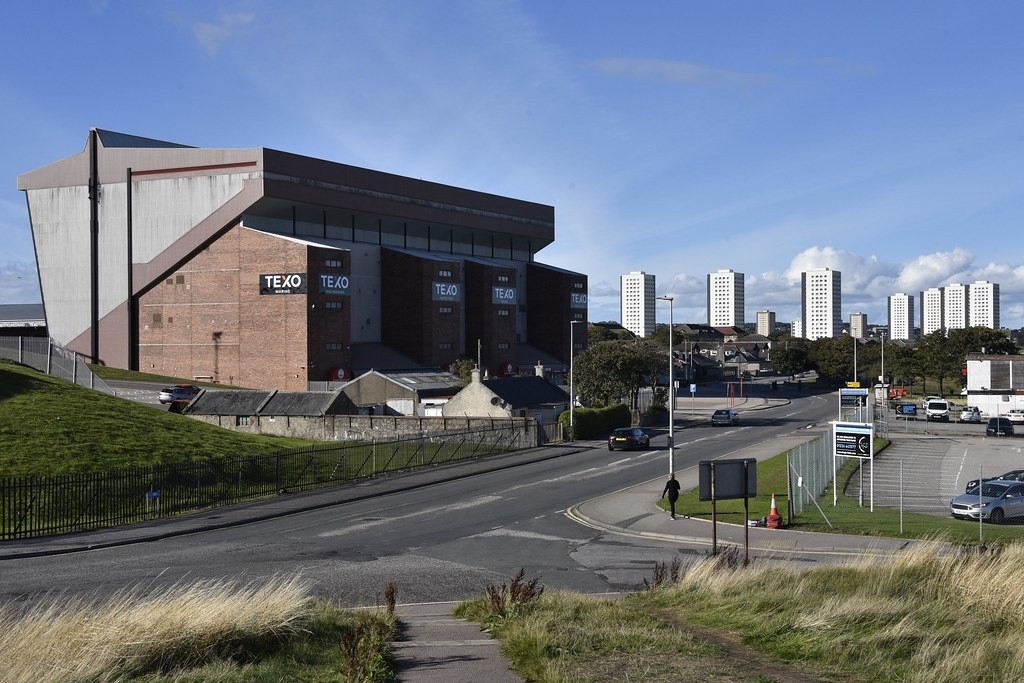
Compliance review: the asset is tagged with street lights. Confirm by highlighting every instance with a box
[570,320,583,426]
[655,295,675,479]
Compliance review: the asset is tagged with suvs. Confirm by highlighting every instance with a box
[175,384,202,395]
[158,386,197,404]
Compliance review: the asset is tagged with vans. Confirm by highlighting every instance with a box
[922,395,940,411]
[927,400,949,423]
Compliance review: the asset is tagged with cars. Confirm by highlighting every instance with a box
[959,406,982,424]
[738,370,752,382]
[986,417,1015,438]
[608,427,650,450]
[1005,409,1024,425]
[948,479,1024,525]
[794,370,811,378]
[965,469,1024,497]
[711,408,739,427]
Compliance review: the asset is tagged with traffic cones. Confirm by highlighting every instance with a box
[766,493,784,529]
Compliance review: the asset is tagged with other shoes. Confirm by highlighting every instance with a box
[670,517,675,520]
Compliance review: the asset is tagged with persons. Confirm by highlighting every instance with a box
[661,473,681,520]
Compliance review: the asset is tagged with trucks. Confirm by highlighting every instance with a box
[894,401,917,421]
